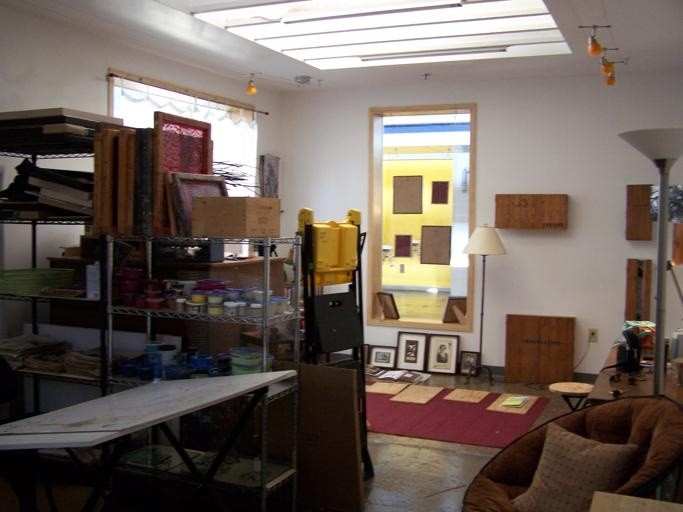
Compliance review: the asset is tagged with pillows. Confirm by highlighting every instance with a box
[508,420,638,510]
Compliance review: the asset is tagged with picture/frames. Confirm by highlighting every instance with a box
[442,297,467,322]
[426,333,459,374]
[368,345,397,369]
[394,332,428,372]
[459,351,480,376]
[377,292,399,319]
[172,172,229,237]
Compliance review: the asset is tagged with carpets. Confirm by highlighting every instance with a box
[364,378,550,450]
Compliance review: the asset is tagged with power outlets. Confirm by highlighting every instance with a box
[588,330,600,342]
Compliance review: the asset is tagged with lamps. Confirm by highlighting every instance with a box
[598,48,619,73]
[242,73,260,94]
[605,61,624,85]
[620,129,683,395]
[462,224,507,385]
[578,25,611,56]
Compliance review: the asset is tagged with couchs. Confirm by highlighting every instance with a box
[464,395,683,512]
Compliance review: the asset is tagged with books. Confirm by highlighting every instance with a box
[0,157,94,219]
[364,363,431,385]
[0,333,129,379]
[664,330,683,360]
[502,398,528,407]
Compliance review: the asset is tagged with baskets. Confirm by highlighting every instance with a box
[0,267,75,298]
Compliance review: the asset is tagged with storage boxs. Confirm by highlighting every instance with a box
[192,196,281,237]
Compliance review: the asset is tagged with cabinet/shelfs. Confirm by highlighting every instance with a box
[1,117,157,512]
[101,233,302,511]
[504,314,574,383]
[495,193,568,230]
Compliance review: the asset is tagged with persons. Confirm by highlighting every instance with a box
[437,345,448,363]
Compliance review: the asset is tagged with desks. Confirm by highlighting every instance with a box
[2,371,296,511]
